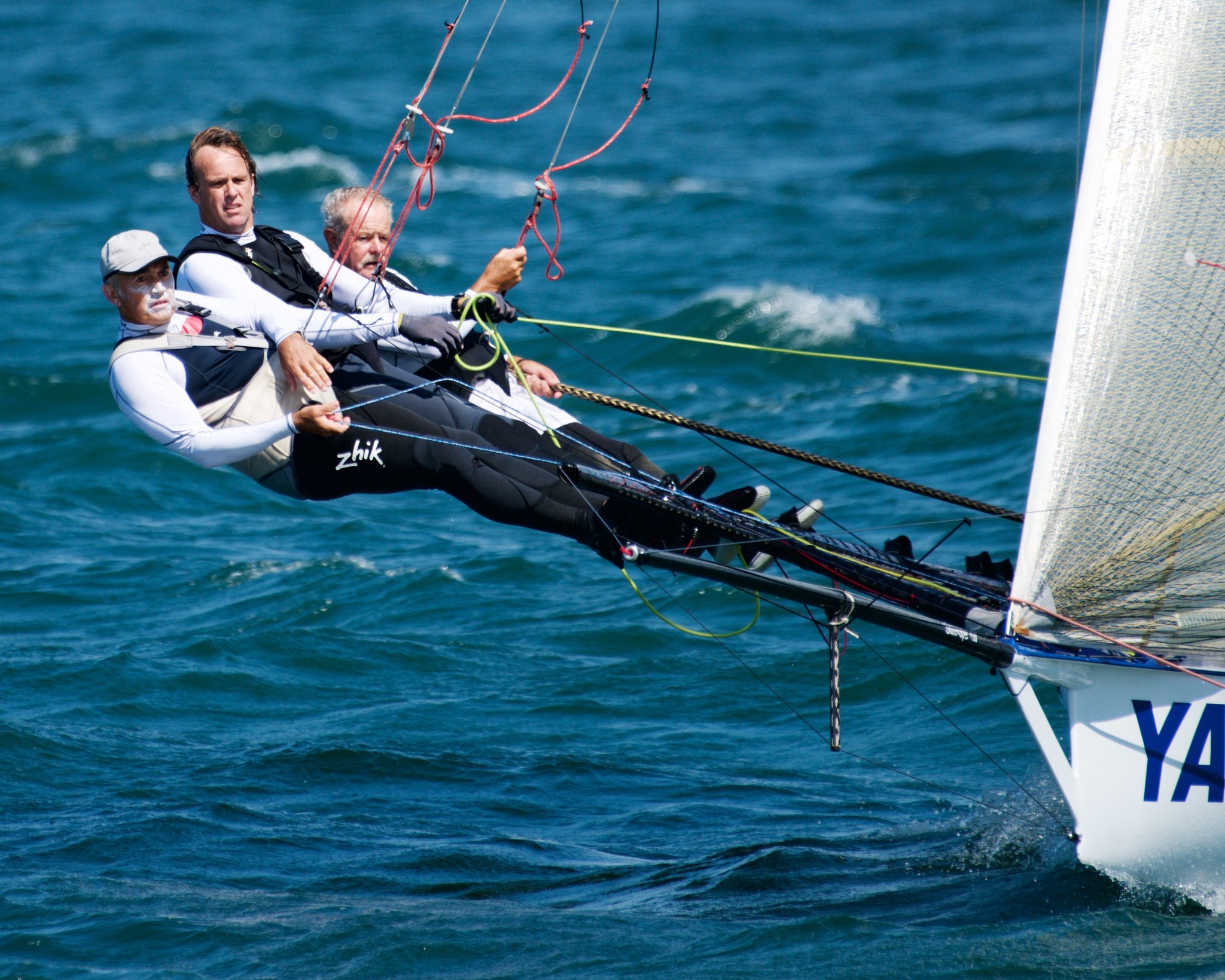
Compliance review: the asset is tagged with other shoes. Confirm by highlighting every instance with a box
[588,467,637,569]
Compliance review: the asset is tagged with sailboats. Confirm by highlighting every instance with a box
[294,0,1225,923]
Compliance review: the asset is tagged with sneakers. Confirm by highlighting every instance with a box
[707,486,771,564]
[738,499,823,573]
[675,486,757,559]
[678,466,715,500]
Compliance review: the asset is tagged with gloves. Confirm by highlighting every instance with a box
[460,292,518,323]
[397,315,464,356]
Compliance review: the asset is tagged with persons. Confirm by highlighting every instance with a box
[173,125,757,559]
[320,185,824,573]
[99,228,680,568]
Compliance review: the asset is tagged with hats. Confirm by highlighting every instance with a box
[100,230,180,283]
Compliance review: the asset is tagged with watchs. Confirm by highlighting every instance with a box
[451,294,471,320]
